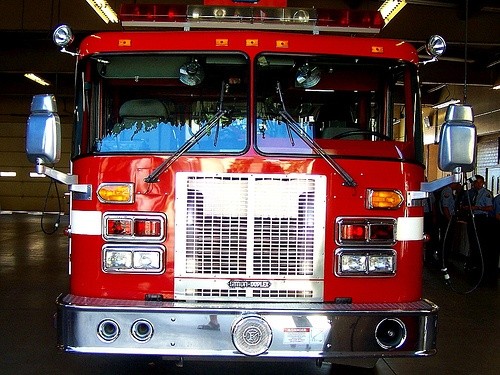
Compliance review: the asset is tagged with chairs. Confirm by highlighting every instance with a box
[118,98,169,125]
[318,106,364,139]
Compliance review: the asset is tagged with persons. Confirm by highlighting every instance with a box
[423,176,436,264]
[464,175,494,283]
[440,183,458,269]
[489,194,500,276]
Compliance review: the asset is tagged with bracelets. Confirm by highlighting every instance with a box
[478,207,480,210]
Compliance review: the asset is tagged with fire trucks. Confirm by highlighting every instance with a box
[26,0,479,369]
